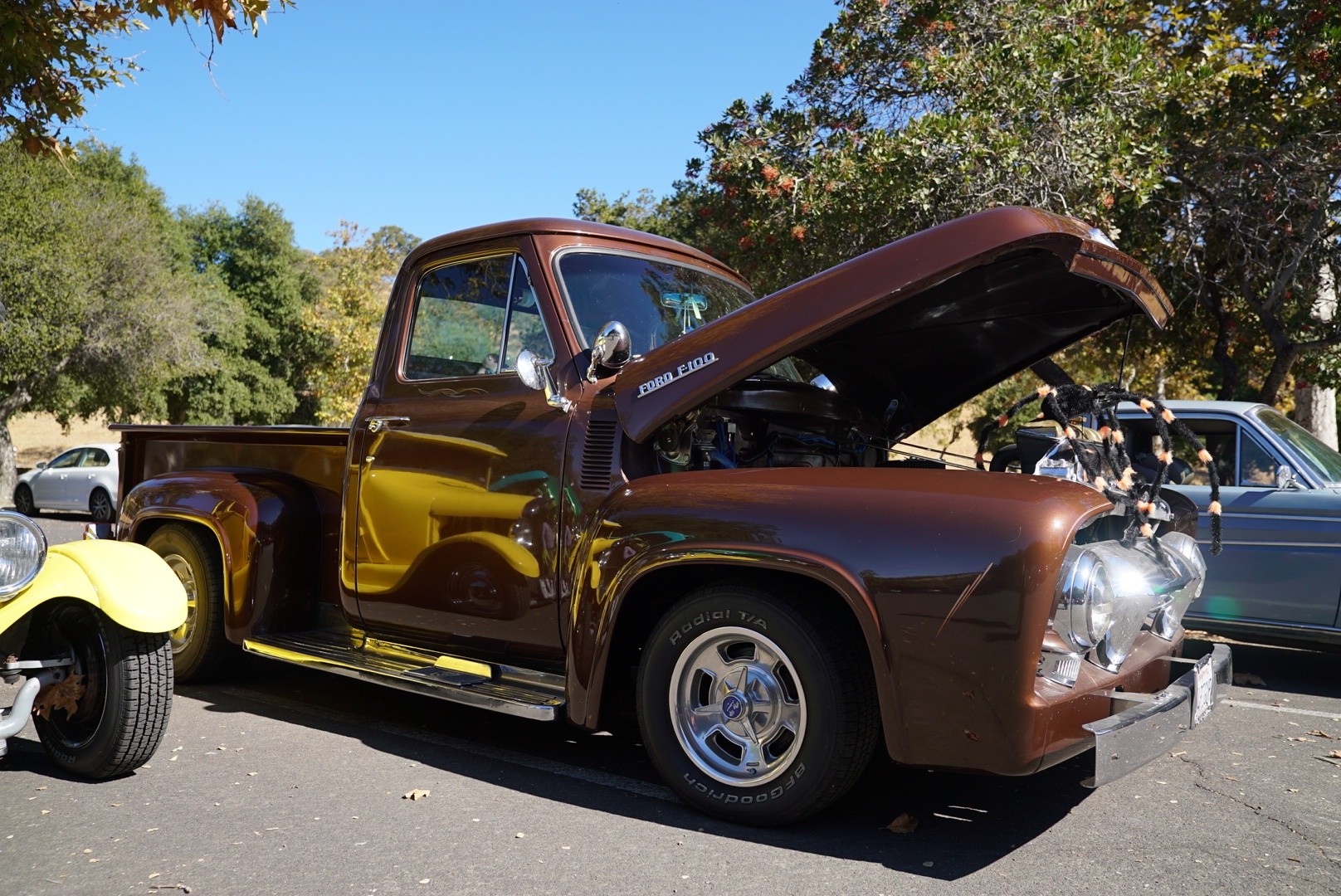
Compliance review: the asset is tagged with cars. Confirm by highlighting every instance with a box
[1,508,181,762]
[12,443,121,523]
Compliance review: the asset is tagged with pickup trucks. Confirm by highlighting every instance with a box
[1038,399,1340,658]
[106,204,1234,829]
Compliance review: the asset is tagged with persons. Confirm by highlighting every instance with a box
[1240,446,1279,487]
